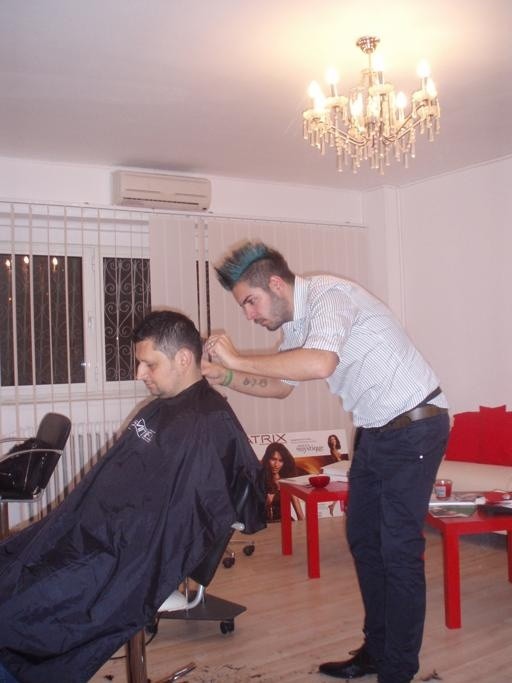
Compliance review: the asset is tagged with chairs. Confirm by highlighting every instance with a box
[127,470,250,683]
[2,413,71,538]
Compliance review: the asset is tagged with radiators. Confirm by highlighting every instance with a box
[18,420,127,528]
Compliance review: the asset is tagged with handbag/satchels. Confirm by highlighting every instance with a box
[2,438,51,500]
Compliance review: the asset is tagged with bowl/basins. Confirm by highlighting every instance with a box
[309,475,330,489]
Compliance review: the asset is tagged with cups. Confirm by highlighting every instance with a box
[433,479,453,501]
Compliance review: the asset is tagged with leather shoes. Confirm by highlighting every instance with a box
[319,648,378,679]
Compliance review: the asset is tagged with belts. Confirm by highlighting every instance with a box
[392,407,448,429]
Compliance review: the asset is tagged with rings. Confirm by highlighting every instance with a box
[210,341,214,346]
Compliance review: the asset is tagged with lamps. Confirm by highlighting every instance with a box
[301,36,444,180]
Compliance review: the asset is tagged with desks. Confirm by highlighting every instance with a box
[424,491,512,630]
[279,480,350,579]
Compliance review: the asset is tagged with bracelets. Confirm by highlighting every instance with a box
[221,369,233,387]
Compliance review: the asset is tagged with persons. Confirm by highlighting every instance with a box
[197,239,450,683]
[0,312,238,683]
[261,442,306,521]
[328,435,350,516]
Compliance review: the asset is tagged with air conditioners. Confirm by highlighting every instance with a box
[109,169,214,212]
